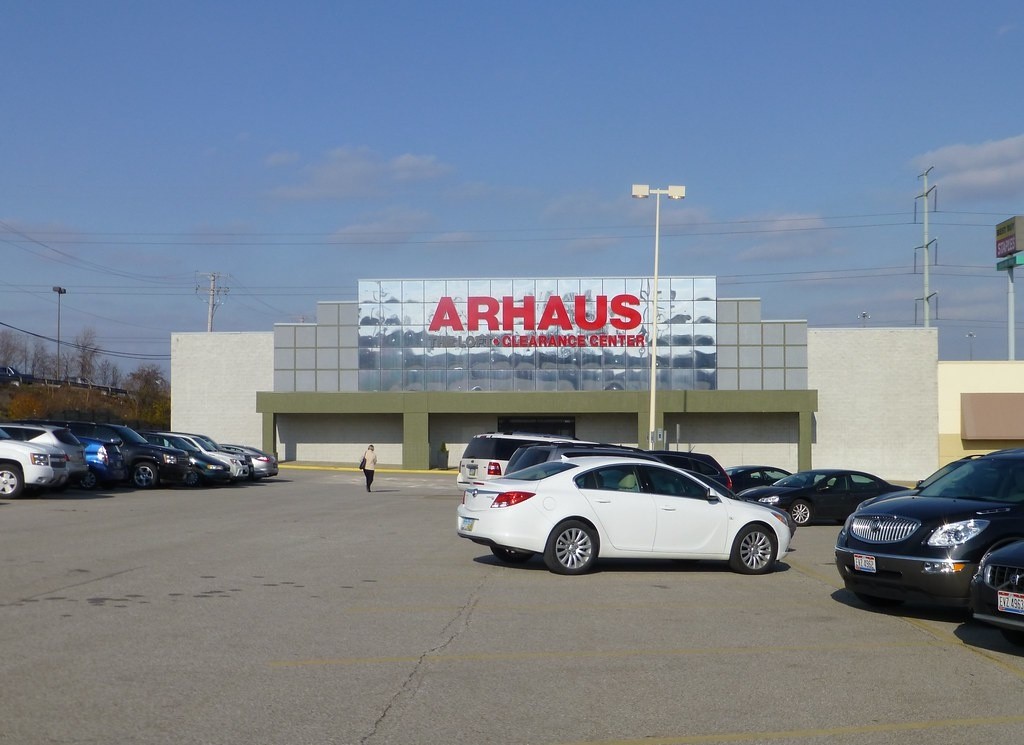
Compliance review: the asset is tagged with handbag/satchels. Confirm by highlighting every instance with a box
[359,457,366,469]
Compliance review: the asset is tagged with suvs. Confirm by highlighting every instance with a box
[22,417,191,488]
[503,440,798,541]
[457,432,601,493]
[637,449,733,496]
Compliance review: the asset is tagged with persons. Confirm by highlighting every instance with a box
[362,445,377,492]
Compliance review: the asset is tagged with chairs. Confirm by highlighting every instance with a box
[618,473,639,493]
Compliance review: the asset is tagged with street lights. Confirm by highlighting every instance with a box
[629,182,687,450]
[53,286,67,380]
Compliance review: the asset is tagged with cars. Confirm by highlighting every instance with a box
[155,431,250,484]
[841,455,1022,610]
[218,444,278,482]
[723,465,793,494]
[76,435,125,490]
[0,439,70,498]
[969,537,1024,646]
[134,431,230,488]
[736,469,912,526]
[854,454,989,512]
[0,365,33,386]
[458,456,792,575]
[1,421,88,490]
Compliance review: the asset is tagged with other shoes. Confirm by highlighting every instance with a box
[366,484,371,492]
